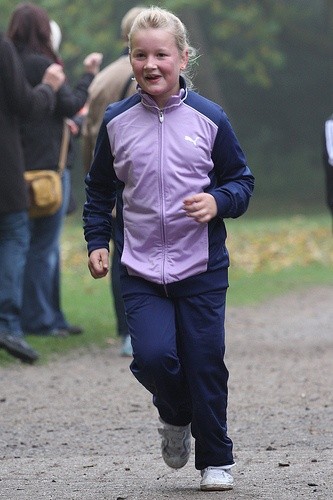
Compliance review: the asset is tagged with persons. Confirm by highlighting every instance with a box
[83,6,254,491]
[322,114,333,214]
[0,6,145,364]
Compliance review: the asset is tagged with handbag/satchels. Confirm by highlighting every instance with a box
[23,169,63,219]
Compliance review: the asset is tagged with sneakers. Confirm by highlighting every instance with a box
[199,467,235,490]
[158,418,193,470]
[0,334,39,364]
[56,325,82,336]
[120,334,133,357]
